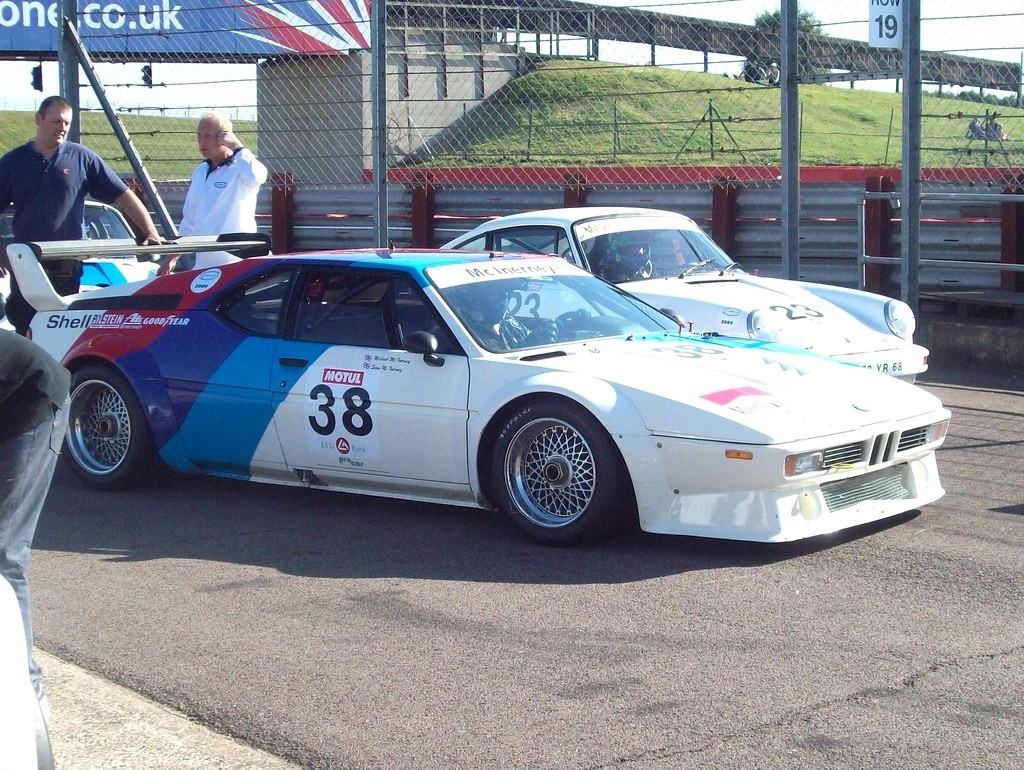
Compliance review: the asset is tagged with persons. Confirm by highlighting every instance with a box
[0,94,184,341]
[456,281,596,354]
[603,229,668,285]
[155,114,268,278]
[737,60,767,84]
[963,118,987,139]
[0,331,74,713]
[765,62,781,87]
[985,118,1004,140]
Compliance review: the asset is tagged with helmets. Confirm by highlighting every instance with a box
[465,286,510,322]
[606,233,651,270]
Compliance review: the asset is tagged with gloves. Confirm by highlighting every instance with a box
[528,318,562,346]
[572,309,593,320]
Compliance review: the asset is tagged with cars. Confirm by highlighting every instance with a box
[0,206,177,336]
[435,206,930,387]
[3,232,951,544]
